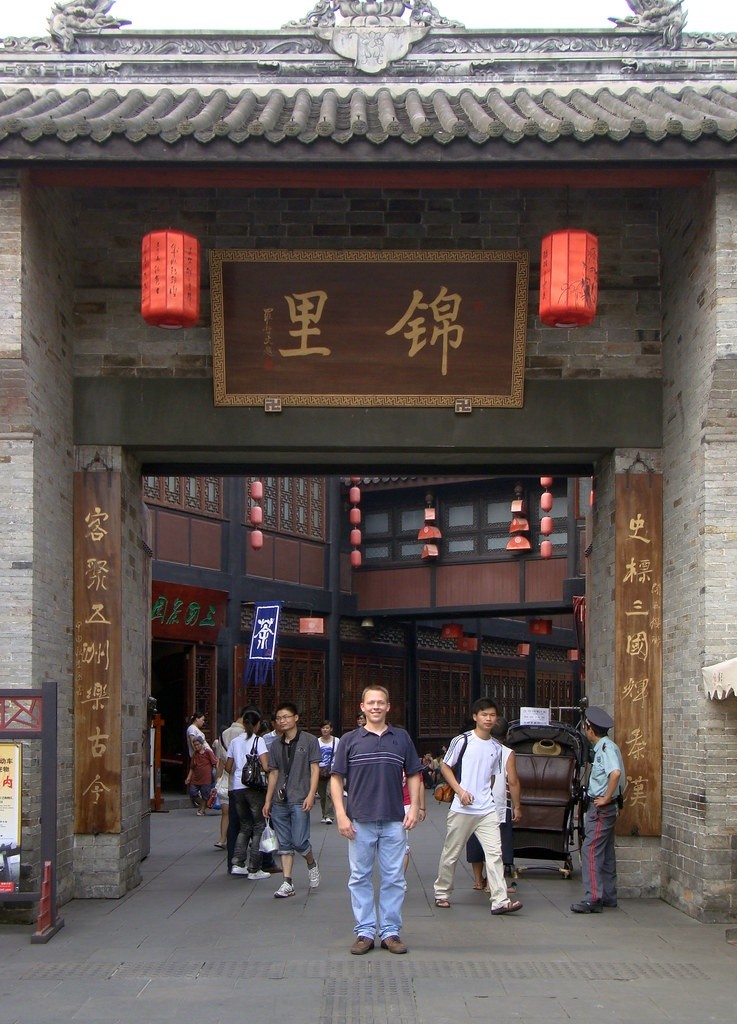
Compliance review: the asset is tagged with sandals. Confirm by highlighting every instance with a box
[491,901,522,915]
[435,898,450,907]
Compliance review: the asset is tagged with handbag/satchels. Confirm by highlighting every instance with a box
[216,773,229,798]
[259,817,280,853]
[433,734,467,802]
[242,736,264,791]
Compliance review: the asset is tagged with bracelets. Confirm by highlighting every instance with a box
[419,808,425,811]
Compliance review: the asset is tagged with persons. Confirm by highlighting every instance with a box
[433,697,523,915]
[178,683,632,955]
[329,685,426,955]
[224,710,271,880]
[184,736,217,816]
[262,702,322,899]
[570,706,626,913]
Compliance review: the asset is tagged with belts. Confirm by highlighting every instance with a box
[589,797,615,805]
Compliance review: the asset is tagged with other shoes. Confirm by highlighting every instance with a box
[403,879,408,891]
[197,809,204,816]
[321,818,325,822]
[325,817,332,823]
[472,877,491,893]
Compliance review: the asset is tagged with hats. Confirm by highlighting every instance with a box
[532,739,561,756]
[585,705,614,732]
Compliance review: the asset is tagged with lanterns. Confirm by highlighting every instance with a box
[515,643,531,658]
[566,649,579,662]
[250,480,264,550]
[441,623,463,638]
[417,504,442,561]
[348,475,364,569]
[139,228,203,330]
[538,476,556,560]
[536,229,602,330]
[505,499,531,553]
[455,637,479,652]
[529,618,554,635]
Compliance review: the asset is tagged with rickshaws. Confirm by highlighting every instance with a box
[503,719,594,878]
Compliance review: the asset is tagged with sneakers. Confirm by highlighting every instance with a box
[231,865,249,874]
[350,936,374,954]
[600,899,618,907]
[307,859,320,888]
[274,881,295,898]
[382,934,407,953]
[264,864,283,872]
[213,841,226,850]
[570,899,603,914]
[247,869,271,879]
